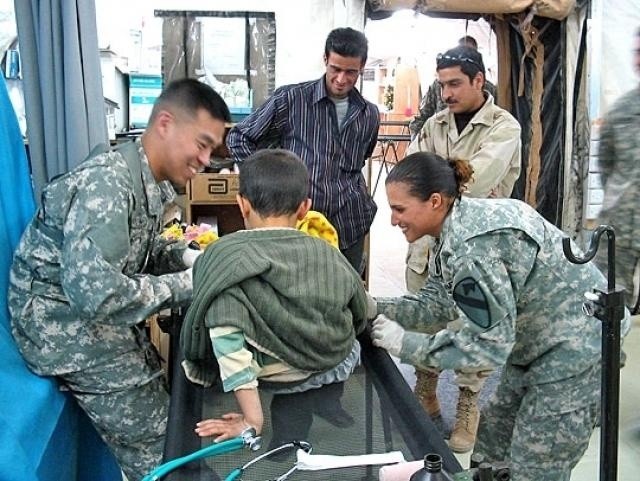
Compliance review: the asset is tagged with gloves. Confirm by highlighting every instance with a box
[371,314,405,358]
[186,267,193,280]
[365,290,377,320]
[183,248,202,267]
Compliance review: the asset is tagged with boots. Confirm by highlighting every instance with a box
[451,392,480,452]
[412,371,441,420]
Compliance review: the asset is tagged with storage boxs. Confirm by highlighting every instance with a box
[192,175,241,202]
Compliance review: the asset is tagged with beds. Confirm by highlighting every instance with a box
[368,134,413,198]
[158,301,468,480]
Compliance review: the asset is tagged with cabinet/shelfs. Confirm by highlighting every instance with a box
[185,174,246,232]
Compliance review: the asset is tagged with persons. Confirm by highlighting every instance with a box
[597,24,640,306]
[405,45,522,453]
[365,152,631,481]
[229,27,380,459]
[411,35,496,131]
[7,78,232,480]
[195,148,360,443]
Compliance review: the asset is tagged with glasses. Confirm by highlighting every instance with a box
[436,52,486,73]
